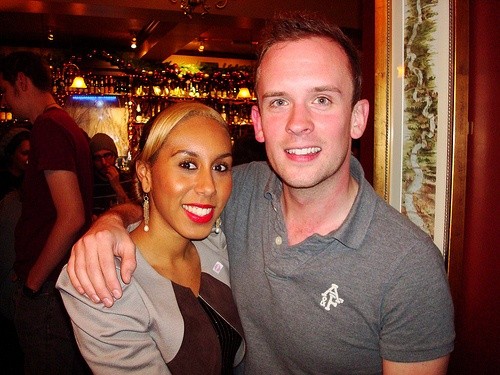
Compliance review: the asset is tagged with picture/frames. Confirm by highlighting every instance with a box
[374,0,470,314]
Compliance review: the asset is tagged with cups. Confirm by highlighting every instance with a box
[113,157,127,171]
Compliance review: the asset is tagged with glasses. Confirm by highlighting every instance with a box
[92,152,112,160]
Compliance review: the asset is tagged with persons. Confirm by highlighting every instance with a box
[55,100,249,375]
[0,51,132,375]
[67,11,454,375]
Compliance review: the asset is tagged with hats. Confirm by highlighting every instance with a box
[0,128,31,159]
[90,133,118,158]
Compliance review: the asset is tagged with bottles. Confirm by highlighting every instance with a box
[49,49,131,68]
[128,124,142,148]
[118,98,169,123]
[51,64,231,98]
[231,125,253,146]
[170,98,252,126]
[231,70,255,97]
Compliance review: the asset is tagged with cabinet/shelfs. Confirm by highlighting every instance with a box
[127,95,258,162]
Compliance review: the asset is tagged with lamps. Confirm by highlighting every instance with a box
[197,40,206,52]
[52,63,88,107]
